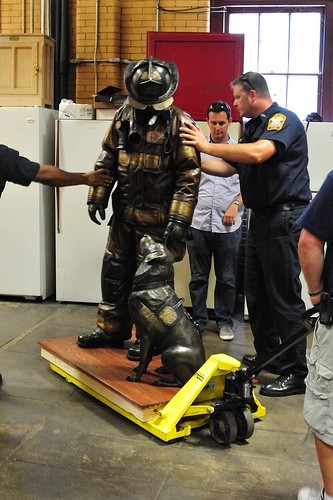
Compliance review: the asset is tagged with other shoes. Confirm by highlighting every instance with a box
[127,339,161,360]
[76,326,127,348]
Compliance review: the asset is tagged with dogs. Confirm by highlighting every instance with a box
[125,233,205,388]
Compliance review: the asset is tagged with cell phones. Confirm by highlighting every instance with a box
[319,292,331,324]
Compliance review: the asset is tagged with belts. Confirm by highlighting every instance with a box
[251,201,309,213]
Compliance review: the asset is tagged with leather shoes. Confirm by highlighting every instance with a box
[258,371,306,397]
[242,354,280,373]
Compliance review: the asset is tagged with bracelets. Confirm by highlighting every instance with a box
[234,200,240,208]
[309,289,323,297]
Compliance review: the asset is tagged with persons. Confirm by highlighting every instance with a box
[296,170,333,500]
[180,71,313,397]
[87,56,201,358]
[185,102,244,340]
[0,143,113,384]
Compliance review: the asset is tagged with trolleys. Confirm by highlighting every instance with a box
[49,305,321,448]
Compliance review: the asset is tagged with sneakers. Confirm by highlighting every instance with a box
[296,486,333,500]
[219,325,235,341]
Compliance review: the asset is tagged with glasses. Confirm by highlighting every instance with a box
[239,71,257,90]
[209,102,231,120]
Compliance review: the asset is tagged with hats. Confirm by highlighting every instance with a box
[122,58,180,105]
